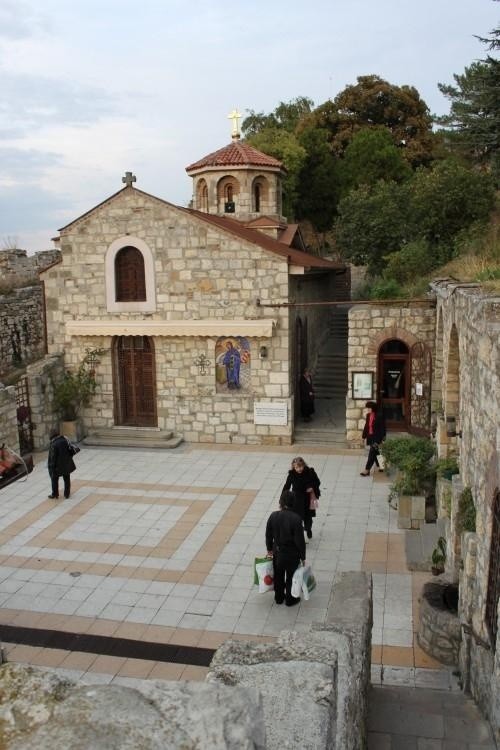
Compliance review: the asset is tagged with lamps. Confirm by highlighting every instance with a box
[445,415,464,439]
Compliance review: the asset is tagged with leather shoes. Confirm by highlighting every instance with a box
[360,472,370,476]
[276,593,285,604]
[306,527,312,538]
[286,597,300,606]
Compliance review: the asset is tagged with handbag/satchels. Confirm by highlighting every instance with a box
[376,452,391,470]
[299,563,317,600]
[63,435,80,455]
[254,554,274,585]
[291,567,305,598]
[256,560,275,594]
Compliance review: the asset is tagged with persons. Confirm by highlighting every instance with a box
[361,400,384,476]
[300,367,314,423]
[280,456,320,539]
[223,341,241,390]
[264,491,307,607]
[48,428,76,499]
[383,362,391,418]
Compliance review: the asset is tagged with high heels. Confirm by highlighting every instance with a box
[48,495,58,498]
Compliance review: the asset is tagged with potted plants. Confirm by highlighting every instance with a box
[431,537,447,576]
[39,346,109,445]
[379,435,437,529]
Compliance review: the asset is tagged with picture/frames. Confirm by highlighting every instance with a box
[351,371,374,400]
